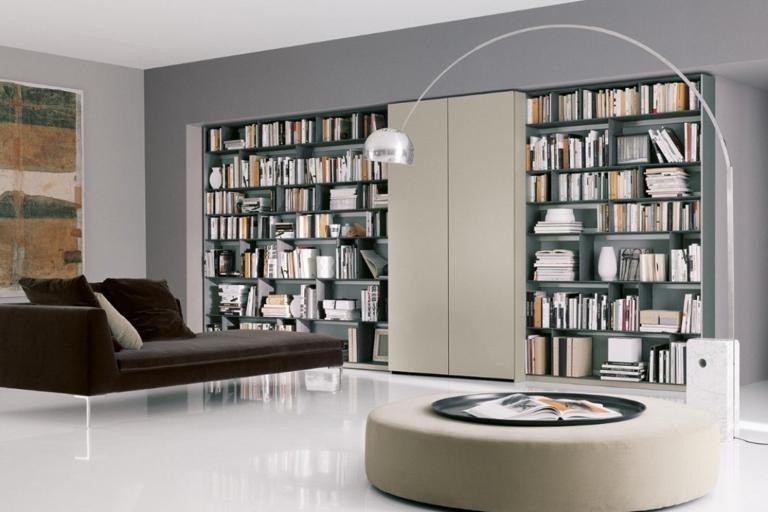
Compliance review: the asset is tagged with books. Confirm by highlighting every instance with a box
[340,339,349,363]
[210,370,302,417]
[600,339,687,386]
[204,111,388,240]
[525,80,699,125]
[525,130,609,203]
[610,122,699,199]
[463,392,622,421]
[208,323,295,331]
[526,291,700,334]
[527,335,593,377]
[204,237,387,322]
[349,328,358,362]
[533,200,701,282]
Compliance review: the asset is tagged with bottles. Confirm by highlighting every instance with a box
[597,247,618,282]
[208,166,222,189]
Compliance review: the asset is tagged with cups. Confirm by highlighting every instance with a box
[330,222,340,238]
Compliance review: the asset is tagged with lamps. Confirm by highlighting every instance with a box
[361,21,740,461]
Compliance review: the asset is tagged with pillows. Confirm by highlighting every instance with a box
[18,274,196,352]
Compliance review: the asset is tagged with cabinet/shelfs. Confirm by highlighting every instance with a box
[526,74,715,391]
[389,89,526,382]
[202,103,389,371]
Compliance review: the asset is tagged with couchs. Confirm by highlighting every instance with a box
[1,303,342,461]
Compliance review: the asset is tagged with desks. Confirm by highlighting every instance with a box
[366,386,719,511]
